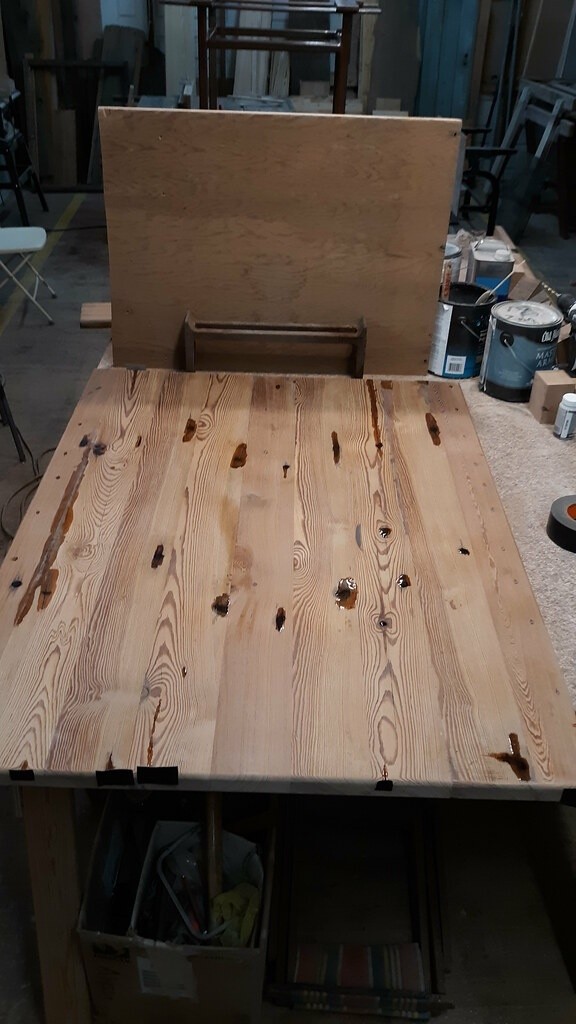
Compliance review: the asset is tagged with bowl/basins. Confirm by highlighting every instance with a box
[441,242,463,283]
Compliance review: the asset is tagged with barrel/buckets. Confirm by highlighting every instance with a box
[480,300,563,403]
[470,236,514,300]
[428,281,497,379]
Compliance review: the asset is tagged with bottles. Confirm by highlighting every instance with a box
[553,393,576,439]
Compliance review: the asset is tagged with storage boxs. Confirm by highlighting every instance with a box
[76,789,281,1024]
[529,370,574,423]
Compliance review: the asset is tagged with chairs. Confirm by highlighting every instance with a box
[0,226,61,327]
